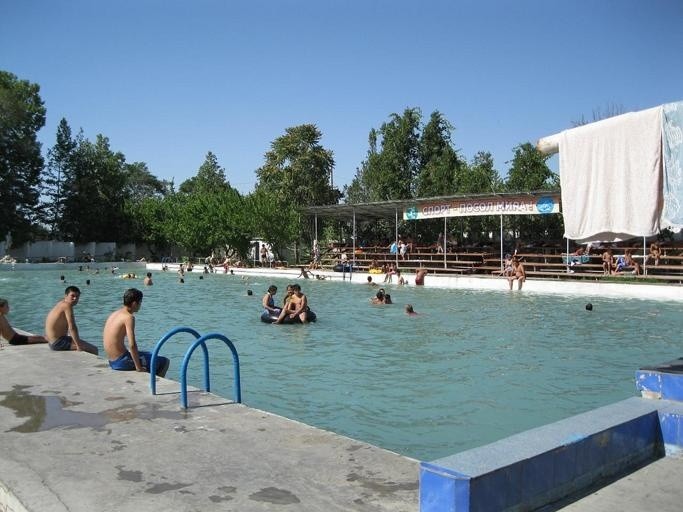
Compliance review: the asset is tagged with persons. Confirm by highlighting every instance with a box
[561,239,662,276]
[0,298,48,345]
[272,283,294,310]
[272,284,309,324]
[45,286,99,355]
[297,267,314,279]
[497,237,536,291]
[313,230,445,317]
[103,289,170,378]
[586,303,593,311]
[60,244,269,297]
[263,285,282,315]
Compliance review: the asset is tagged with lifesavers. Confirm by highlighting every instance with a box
[333,265,350,272]
[369,268,382,273]
[261,308,316,324]
